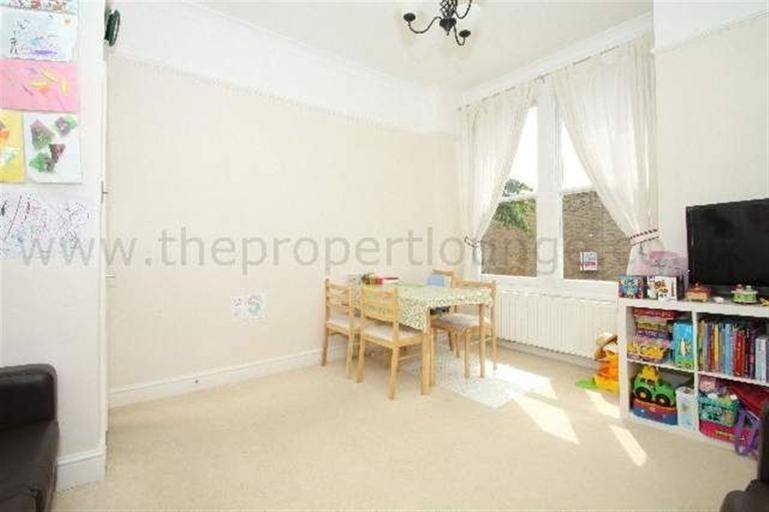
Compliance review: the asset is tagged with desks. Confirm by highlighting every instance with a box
[350,283,492,398]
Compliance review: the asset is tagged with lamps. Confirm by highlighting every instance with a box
[401,0,483,46]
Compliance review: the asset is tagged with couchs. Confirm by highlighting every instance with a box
[0,364,61,512]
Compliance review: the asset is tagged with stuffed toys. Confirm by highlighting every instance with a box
[732,283,759,304]
[633,366,676,406]
[687,282,711,302]
[575,340,619,394]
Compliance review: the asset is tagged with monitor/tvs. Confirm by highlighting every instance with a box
[685,198,769,299]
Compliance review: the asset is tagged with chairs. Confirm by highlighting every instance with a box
[322,277,372,378]
[355,285,434,399]
[426,267,499,379]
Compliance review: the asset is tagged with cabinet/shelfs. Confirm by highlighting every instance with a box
[619,296,769,458]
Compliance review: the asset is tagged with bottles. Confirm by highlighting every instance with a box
[364,271,376,285]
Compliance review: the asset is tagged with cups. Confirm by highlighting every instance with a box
[376,278,383,285]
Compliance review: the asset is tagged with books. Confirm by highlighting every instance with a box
[627,307,690,364]
[698,316,768,384]
